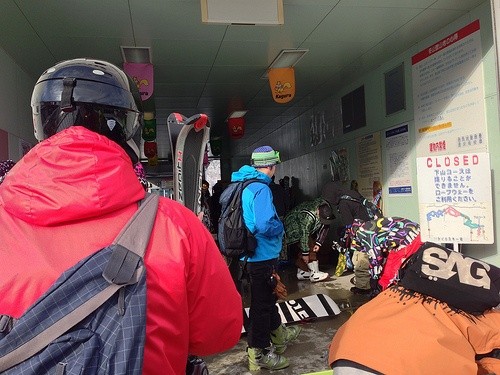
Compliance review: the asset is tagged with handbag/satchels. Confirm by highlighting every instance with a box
[0,190,159,375]
[397,241,500,314]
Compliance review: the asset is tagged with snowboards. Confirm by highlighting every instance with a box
[241,294,341,334]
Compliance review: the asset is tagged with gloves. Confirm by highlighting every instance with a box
[272,273,287,299]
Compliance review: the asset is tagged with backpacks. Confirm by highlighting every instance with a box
[218,179,268,258]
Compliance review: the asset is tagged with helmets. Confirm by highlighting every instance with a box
[31,58,139,145]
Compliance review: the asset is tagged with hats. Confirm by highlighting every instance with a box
[251,146,281,167]
[319,204,335,224]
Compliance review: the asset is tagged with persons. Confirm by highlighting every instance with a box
[328,242,500,375]
[332,188,372,277]
[282,197,335,282]
[345,216,422,293]
[238,144,301,375]
[0,57,244,375]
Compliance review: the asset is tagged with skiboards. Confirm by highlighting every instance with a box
[166,111,212,374]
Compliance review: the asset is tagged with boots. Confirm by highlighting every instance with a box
[271,323,302,355]
[298,270,310,280]
[310,261,328,281]
[248,346,289,372]
